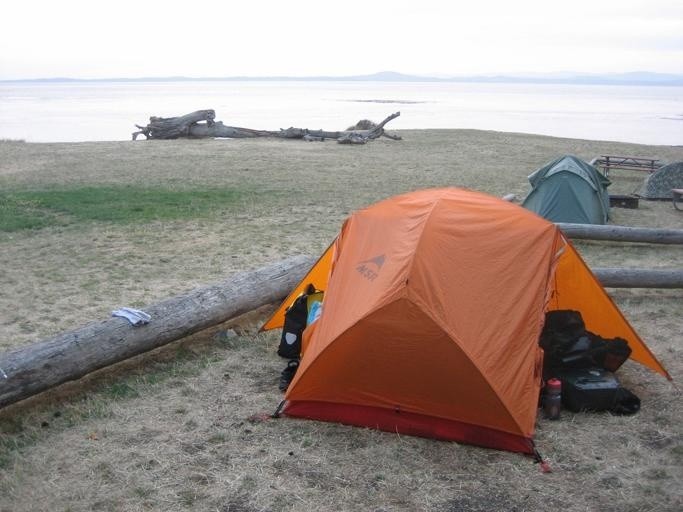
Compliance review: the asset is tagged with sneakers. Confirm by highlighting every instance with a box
[280,361,298,392]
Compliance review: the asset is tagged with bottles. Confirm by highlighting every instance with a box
[544,376,562,421]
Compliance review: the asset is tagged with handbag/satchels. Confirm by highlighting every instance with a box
[277,283,324,358]
[563,367,623,412]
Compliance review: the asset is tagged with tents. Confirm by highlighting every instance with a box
[519,153,614,226]
[630,161,683,201]
[254,185,676,477]
